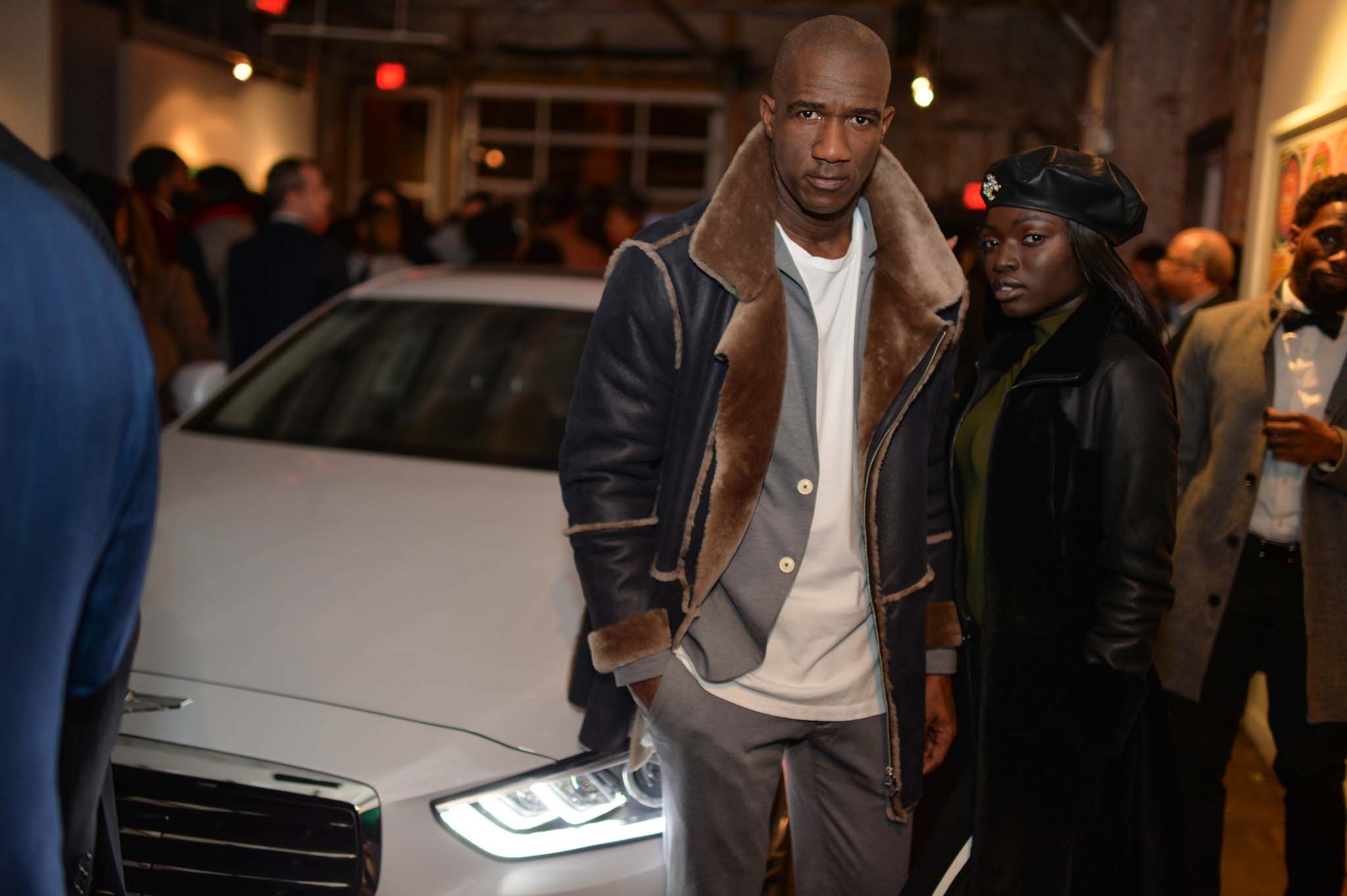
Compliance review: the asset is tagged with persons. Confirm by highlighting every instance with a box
[558,15,981,896]
[0,125,160,896]
[1173,175,1347,896]
[943,147,1179,896]
[122,148,1235,419]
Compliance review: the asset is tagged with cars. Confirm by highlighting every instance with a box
[91,265,791,896]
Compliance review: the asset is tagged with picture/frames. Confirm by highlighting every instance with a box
[1248,91,1347,296]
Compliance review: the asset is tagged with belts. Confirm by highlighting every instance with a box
[1243,534,1306,565]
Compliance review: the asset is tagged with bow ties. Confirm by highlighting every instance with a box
[1280,310,1345,341]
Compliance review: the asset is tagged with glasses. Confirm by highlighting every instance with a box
[1159,248,1197,273]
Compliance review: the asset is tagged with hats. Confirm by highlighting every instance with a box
[979,145,1147,248]
